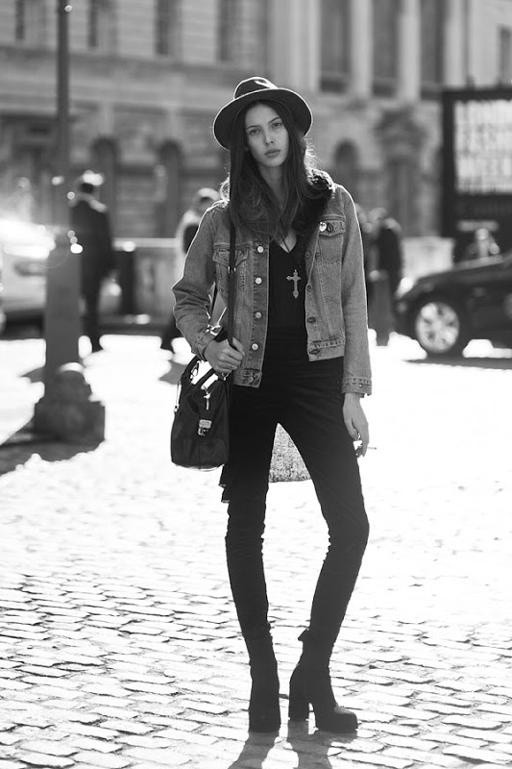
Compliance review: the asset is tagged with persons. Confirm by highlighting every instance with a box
[68,170,114,349]
[172,77,372,733]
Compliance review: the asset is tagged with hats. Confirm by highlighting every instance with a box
[208,72,313,152]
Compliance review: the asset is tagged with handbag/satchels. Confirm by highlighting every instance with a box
[165,347,236,475]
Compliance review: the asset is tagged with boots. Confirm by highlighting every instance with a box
[285,627,362,734]
[238,621,287,736]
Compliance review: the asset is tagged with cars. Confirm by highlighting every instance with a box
[0,219,124,330]
[390,252,512,361]
